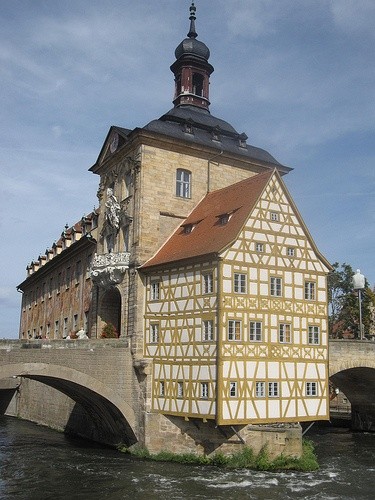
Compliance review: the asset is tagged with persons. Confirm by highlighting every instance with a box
[99,188,121,253]
[352,269,365,289]
[28,332,77,340]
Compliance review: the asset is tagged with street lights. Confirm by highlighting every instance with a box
[352,269,365,340]
